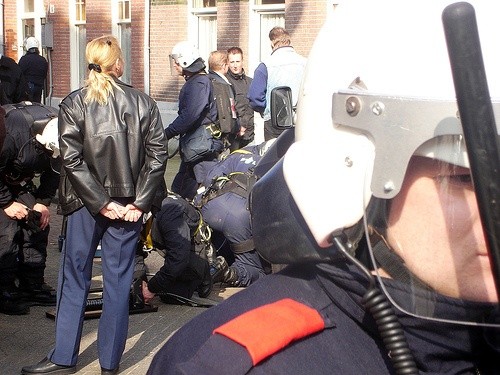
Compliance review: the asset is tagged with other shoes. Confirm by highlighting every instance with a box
[18,278,57,305]
[0,280,30,315]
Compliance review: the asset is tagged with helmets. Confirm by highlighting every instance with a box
[25,37,39,51]
[169,42,206,68]
[36,117,60,158]
[250,1,500,265]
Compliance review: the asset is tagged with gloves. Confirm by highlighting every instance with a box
[26,210,50,236]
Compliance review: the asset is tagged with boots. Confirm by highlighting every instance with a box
[210,256,234,286]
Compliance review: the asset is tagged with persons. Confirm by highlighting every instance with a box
[0,27,306,316]
[144,0,500,375]
[19,36,168,375]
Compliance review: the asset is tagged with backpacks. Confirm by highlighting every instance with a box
[212,83,232,133]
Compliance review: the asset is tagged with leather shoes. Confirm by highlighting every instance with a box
[101,365,119,375]
[199,262,211,297]
[22,356,76,375]
